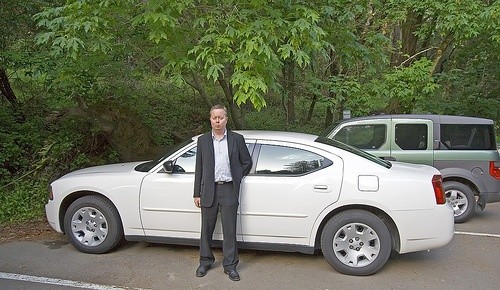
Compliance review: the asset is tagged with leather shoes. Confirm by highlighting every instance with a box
[196,265,211,276]
[224,269,240,281]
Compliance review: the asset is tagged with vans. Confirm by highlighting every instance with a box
[316,115,500,224]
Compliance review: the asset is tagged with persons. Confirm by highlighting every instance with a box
[193,104,253,282]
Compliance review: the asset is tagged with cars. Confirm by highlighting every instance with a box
[44,130,454,277]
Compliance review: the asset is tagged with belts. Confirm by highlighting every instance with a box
[214,181,233,185]
